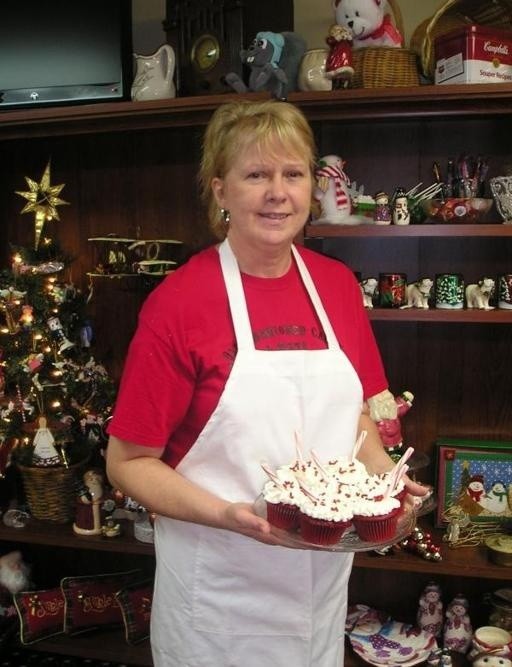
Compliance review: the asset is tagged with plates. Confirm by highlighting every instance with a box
[348,620,438,667]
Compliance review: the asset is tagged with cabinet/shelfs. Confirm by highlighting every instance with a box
[0,82,512,666]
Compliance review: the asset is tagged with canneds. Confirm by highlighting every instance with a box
[379,273,406,307]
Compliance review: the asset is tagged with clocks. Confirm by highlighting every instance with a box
[160,2,294,98]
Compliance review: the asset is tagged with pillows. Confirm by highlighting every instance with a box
[14,569,154,646]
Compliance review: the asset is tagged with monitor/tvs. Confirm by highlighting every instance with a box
[0,0,133,108]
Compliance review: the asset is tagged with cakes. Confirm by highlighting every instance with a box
[257,429,415,547]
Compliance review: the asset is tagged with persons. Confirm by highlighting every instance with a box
[107,98,431,667]
[367,388,413,450]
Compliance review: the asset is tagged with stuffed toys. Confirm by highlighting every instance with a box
[335,1,403,50]
[227,30,309,94]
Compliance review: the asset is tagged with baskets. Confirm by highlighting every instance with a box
[335,1,421,88]
[16,453,92,525]
